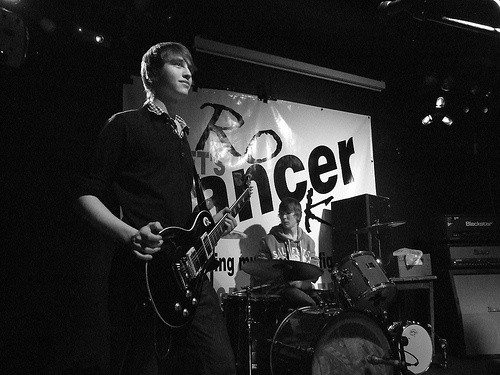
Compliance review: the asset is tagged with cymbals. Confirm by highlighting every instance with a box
[242,258,324,282]
[350,221,406,234]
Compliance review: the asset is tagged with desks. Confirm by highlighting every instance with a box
[396,275,437,356]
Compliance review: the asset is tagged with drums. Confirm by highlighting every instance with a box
[332,251,397,315]
[270,305,400,375]
[388,322,433,374]
[220,291,282,371]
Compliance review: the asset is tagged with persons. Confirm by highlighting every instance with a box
[66,41,238,375]
[260,196,320,305]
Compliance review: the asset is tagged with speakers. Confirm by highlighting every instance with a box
[331,194,393,271]
[435,268,500,358]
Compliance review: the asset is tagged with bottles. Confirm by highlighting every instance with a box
[439,339,448,368]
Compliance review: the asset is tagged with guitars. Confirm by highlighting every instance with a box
[137,175,254,327]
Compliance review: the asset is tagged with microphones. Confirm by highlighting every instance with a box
[375,0,426,21]
[366,355,411,367]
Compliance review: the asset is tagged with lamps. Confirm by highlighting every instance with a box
[479,104,488,114]
[441,79,451,91]
[441,112,454,126]
[417,111,432,125]
[433,95,445,108]
[462,106,469,114]
